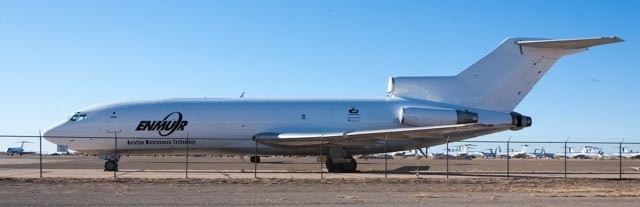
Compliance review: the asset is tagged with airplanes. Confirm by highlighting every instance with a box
[42,32,627,174]
[395,144,640,159]
[0,141,36,157]
